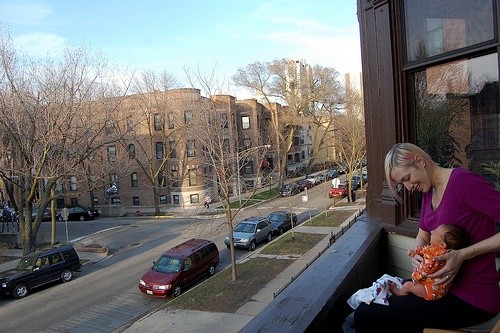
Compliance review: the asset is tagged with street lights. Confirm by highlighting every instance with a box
[237,145,271,205]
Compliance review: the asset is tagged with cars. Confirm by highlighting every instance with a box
[0,205,99,222]
[279,160,367,199]
[266,210,297,236]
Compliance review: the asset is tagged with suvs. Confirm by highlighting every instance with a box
[224,216,273,252]
[0,243,81,300]
[138,237,221,301]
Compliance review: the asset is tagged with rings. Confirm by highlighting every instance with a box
[450,276,455,280]
[445,275,451,279]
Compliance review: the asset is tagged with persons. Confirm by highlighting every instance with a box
[206,199,209,208]
[354,143,500,333]
[389,223,474,301]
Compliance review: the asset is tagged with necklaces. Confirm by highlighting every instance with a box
[432,204,437,209]
